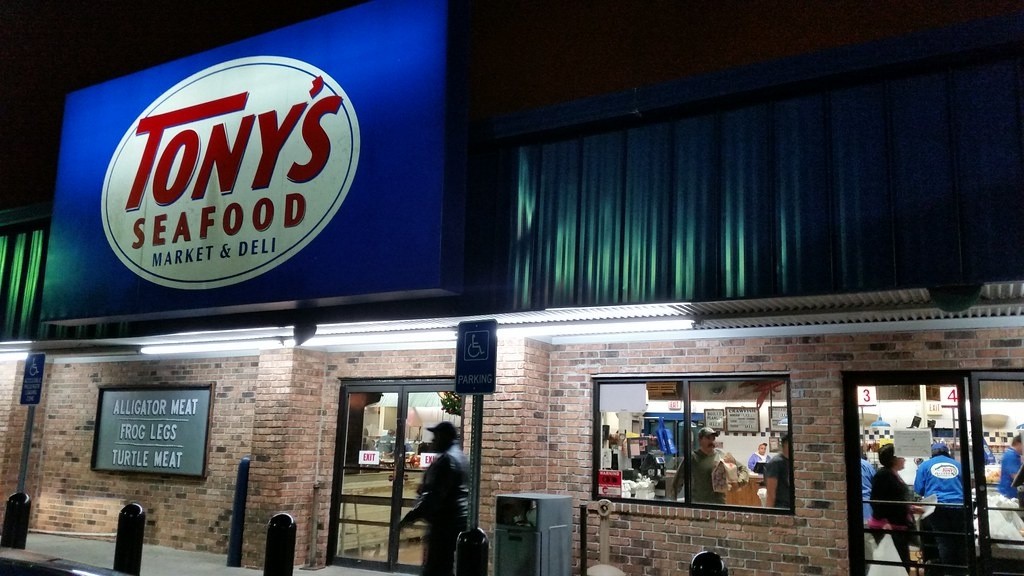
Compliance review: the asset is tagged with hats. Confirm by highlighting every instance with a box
[696,427,720,438]
[423,421,458,438]
[930,441,950,452]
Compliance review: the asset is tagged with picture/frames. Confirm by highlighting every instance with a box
[705,409,725,431]
[726,407,760,433]
[769,407,789,432]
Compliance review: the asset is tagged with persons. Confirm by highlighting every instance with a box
[861,458,877,525]
[765,434,790,509]
[363,427,396,456]
[748,443,771,486]
[912,443,968,576]
[670,427,749,504]
[399,422,469,576]
[870,443,920,575]
[997,435,1022,498]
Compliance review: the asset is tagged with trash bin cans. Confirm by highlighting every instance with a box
[493,493,573,576]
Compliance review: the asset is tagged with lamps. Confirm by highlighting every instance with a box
[871,417,890,427]
